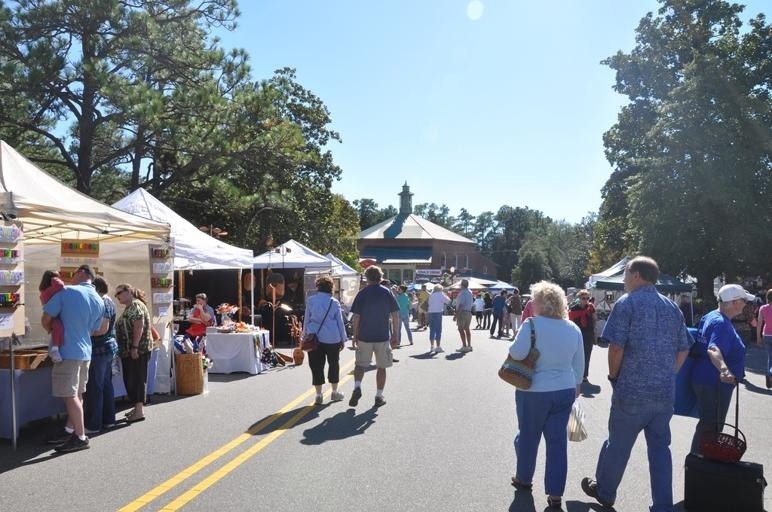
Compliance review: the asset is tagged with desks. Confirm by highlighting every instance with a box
[207,330,270,375]
[1,345,159,450]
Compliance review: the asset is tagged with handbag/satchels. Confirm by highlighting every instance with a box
[498,348,540,390]
[675,327,702,419]
[302,334,320,352]
[151,326,160,342]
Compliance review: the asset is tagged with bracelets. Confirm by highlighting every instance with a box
[607,374,617,382]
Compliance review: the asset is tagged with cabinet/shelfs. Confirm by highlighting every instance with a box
[1,224,25,308]
[149,243,174,305]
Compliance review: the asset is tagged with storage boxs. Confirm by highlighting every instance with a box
[14,349,53,367]
[1,352,38,369]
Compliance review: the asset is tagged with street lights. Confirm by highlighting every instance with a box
[450,266,456,302]
[200,224,222,237]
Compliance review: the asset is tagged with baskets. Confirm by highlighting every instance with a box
[175,353,203,395]
[699,424,746,463]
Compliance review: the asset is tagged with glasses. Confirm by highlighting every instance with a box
[115,290,122,297]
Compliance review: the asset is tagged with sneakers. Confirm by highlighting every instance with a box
[315,387,386,406]
[48,408,145,453]
[48,349,62,363]
[430,345,473,356]
[512,475,615,508]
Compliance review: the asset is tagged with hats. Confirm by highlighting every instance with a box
[718,284,756,302]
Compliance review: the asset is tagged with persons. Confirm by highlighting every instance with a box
[39,264,215,453]
[580,256,689,510]
[509,280,585,511]
[380,279,519,362]
[521,299,536,325]
[745,287,772,389]
[303,275,348,405]
[687,283,756,456]
[349,266,401,406]
[566,289,597,382]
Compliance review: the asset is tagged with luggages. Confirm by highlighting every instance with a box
[686,378,767,512]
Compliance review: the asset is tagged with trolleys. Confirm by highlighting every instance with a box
[703,374,748,462]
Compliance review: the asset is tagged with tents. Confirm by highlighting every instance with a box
[304,253,361,313]
[587,258,693,328]
